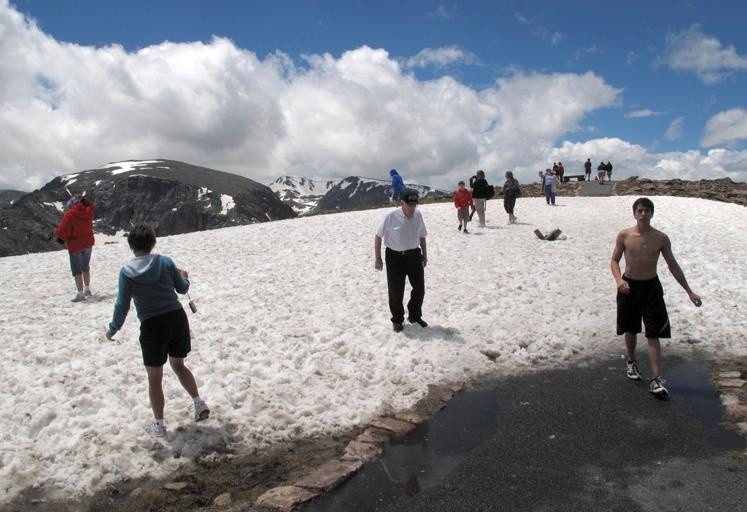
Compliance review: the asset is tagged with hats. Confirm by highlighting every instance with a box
[477,170,485,178]
[399,188,418,205]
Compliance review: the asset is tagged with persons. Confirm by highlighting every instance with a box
[104,221,210,438]
[389,168,406,208]
[470,170,489,228]
[454,181,473,234]
[609,197,703,395]
[53,193,96,303]
[538,162,564,206]
[583,158,613,185]
[496,170,519,225]
[374,187,428,332]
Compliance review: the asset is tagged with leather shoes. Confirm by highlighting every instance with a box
[408,317,427,327]
[393,321,403,332]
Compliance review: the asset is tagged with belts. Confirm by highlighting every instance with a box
[394,249,415,256]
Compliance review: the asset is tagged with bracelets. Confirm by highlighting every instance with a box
[375,256,381,260]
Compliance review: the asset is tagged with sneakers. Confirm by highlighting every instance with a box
[648,377,670,399]
[194,401,210,422]
[625,359,642,381]
[458,224,462,231]
[71,290,86,302]
[83,290,91,297]
[464,229,467,233]
[148,423,167,436]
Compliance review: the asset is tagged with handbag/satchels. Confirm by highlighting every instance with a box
[485,184,494,200]
[515,188,523,197]
